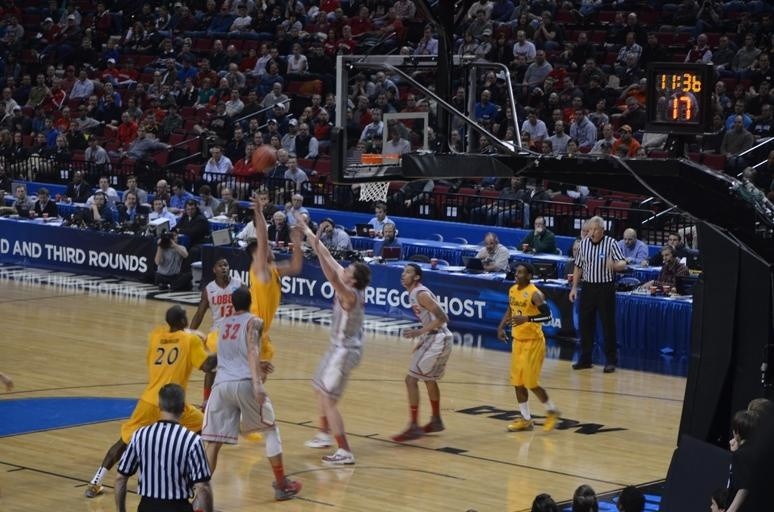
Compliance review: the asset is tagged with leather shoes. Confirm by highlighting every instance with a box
[603,363,615,372]
[572,360,592,370]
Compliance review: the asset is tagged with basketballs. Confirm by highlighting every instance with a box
[250,145,278,173]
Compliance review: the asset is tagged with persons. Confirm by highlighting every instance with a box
[215,188,238,217]
[315,218,353,251]
[474,232,511,272]
[0,371,14,391]
[572,219,589,258]
[367,203,395,238]
[260,190,279,220]
[616,486,645,512]
[497,177,552,230]
[288,208,371,464]
[191,286,303,511]
[572,484,599,512]
[122,175,147,204]
[235,212,270,248]
[373,223,404,262]
[724,410,759,512]
[0,1,774,191]
[391,263,453,442]
[710,489,730,512]
[642,245,689,292]
[617,228,648,265]
[748,398,772,417]
[517,216,556,254]
[154,179,169,206]
[653,232,692,269]
[385,179,434,209]
[115,382,214,512]
[497,262,561,432]
[679,225,698,250]
[153,228,192,291]
[185,192,302,441]
[531,493,559,512]
[11,184,32,213]
[29,187,58,218]
[83,305,217,499]
[189,258,246,413]
[300,214,317,242]
[95,176,120,203]
[283,193,310,224]
[170,179,193,209]
[268,212,289,244]
[120,191,149,224]
[149,197,176,231]
[568,215,629,374]
[199,185,220,211]
[172,199,208,241]
[91,192,112,224]
[63,171,89,204]
[469,177,524,226]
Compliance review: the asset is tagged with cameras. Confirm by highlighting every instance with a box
[160,228,173,248]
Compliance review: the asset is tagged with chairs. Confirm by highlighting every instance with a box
[298,158,314,171]
[185,135,200,152]
[445,188,478,207]
[195,109,208,122]
[169,134,184,147]
[579,200,606,217]
[309,160,333,192]
[704,154,725,170]
[472,191,501,206]
[137,56,153,64]
[431,185,449,216]
[543,195,573,213]
[185,120,198,133]
[428,234,443,242]
[242,40,257,54]
[438,259,449,266]
[153,150,168,166]
[140,74,153,84]
[604,201,632,237]
[228,39,242,49]
[181,106,195,120]
[190,39,211,53]
[453,237,467,244]
[550,0,773,154]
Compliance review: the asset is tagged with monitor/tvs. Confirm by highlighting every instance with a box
[532,261,558,284]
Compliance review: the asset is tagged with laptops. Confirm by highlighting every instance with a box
[355,224,373,237]
[383,247,400,261]
[200,206,214,219]
[461,256,485,273]
[212,229,232,246]
[15,204,30,218]
[108,196,120,210]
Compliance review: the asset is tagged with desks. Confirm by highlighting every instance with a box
[0,215,187,284]
[4,192,245,234]
[350,234,699,296]
[199,243,696,376]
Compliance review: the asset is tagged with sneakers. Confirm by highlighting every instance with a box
[319,448,356,465]
[240,430,265,441]
[393,426,424,443]
[423,415,445,433]
[272,476,302,502]
[541,408,561,433]
[85,480,104,498]
[506,417,535,433]
[304,430,332,448]
